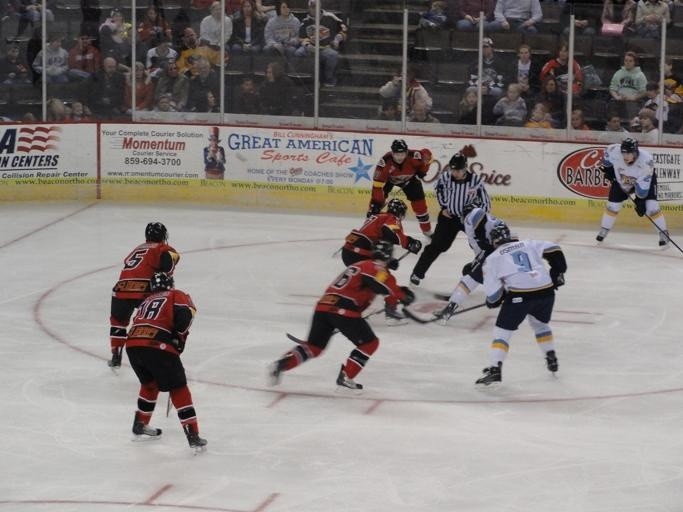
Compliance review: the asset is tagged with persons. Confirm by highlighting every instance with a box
[492,84,527,126]
[301,0,349,87]
[379,68,432,121]
[557,1,604,66]
[48,99,71,122]
[605,52,648,115]
[534,77,566,128]
[493,1,543,44]
[466,39,504,94]
[434,216,568,387]
[454,0,503,37]
[63,102,90,122]
[376,102,398,121]
[232,80,267,114]
[539,42,582,101]
[508,43,538,96]
[592,137,668,250]
[232,0,263,73]
[85,58,128,120]
[153,98,175,113]
[1,47,31,84]
[106,221,181,368]
[97,10,139,60]
[139,6,175,51]
[432,202,510,327]
[645,57,683,133]
[174,28,230,74]
[641,82,668,133]
[366,139,437,237]
[342,199,422,321]
[124,62,153,112]
[264,0,300,66]
[529,102,553,127]
[125,271,208,447]
[183,57,219,112]
[69,33,104,75]
[193,87,217,113]
[199,1,232,49]
[604,113,629,132]
[639,108,659,133]
[571,108,590,129]
[457,90,480,125]
[155,59,192,112]
[261,60,310,116]
[113,24,148,66]
[639,1,671,35]
[4,65,31,84]
[267,250,416,390]
[408,153,491,287]
[32,34,71,85]
[409,102,441,123]
[602,1,637,35]
[203,126,227,180]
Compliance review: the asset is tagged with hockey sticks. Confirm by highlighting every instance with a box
[165,391,173,417]
[401,303,488,324]
[284,299,403,345]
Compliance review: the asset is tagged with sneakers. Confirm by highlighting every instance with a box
[108,349,119,366]
[189,436,209,446]
[433,302,459,320]
[423,230,432,236]
[338,371,361,389]
[386,306,403,318]
[477,363,502,384]
[658,232,667,245]
[132,423,159,437]
[410,273,420,283]
[596,229,607,241]
[545,351,559,372]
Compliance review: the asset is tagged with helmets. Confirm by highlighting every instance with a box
[386,199,405,212]
[485,220,509,248]
[391,140,407,152]
[149,272,173,290]
[369,242,393,261]
[144,223,167,242]
[620,139,637,151]
[449,153,467,167]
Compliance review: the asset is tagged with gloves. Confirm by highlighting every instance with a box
[486,300,501,309]
[549,271,564,288]
[407,238,421,253]
[462,263,473,275]
[415,171,427,178]
[635,197,647,215]
[603,168,613,181]
